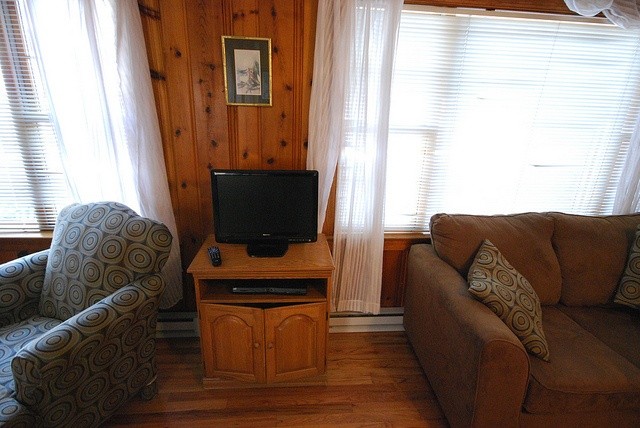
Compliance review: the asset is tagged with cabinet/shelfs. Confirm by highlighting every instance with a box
[187,233,336,384]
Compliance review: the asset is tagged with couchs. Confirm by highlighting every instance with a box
[1,202,172,427]
[402,211,640,427]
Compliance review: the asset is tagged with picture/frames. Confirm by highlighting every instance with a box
[220,35,273,108]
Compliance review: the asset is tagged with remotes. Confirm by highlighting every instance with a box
[207,246,222,267]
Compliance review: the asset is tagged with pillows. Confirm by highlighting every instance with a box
[546,211,640,310]
[427,211,562,305]
[614,224,639,305]
[467,244,550,361]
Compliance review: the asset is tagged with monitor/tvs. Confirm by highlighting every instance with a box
[210,169,319,258]
